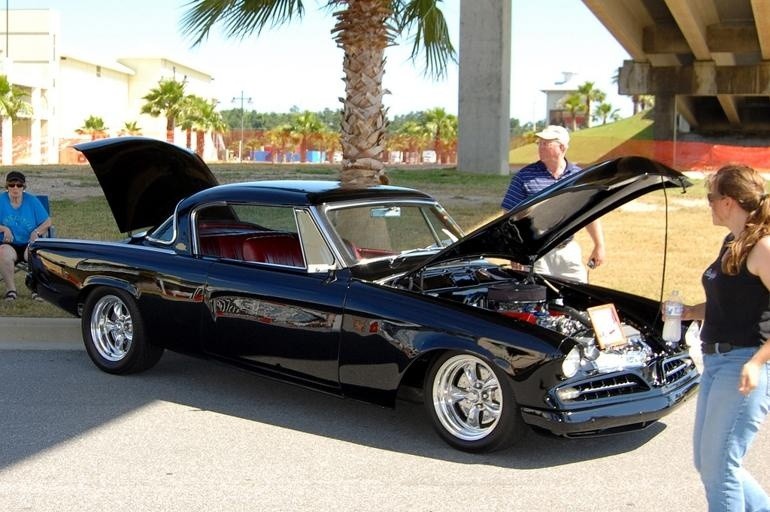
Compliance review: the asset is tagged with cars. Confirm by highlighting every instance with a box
[22,135,703,454]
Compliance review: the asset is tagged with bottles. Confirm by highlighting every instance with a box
[661,290,683,343]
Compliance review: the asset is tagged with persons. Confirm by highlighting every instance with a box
[501,124,604,283]
[661,163,770,511]
[0,171,53,302]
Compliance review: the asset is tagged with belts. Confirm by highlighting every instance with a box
[701,342,732,354]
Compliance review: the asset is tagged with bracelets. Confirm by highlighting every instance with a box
[34,229,40,236]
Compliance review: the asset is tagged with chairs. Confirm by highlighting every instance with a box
[233,233,305,268]
[0,195,50,282]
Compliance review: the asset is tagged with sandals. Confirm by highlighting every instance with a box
[31,292,41,301]
[3,290,17,300]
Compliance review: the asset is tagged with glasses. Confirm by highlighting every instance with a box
[8,183,23,187]
[536,141,553,147]
[706,192,726,204]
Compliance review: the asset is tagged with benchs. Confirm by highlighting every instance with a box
[200,231,282,260]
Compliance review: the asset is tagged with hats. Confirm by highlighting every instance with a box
[6,171,25,183]
[533,125,570,146]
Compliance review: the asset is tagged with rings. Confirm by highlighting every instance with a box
[6,238,8,241]
